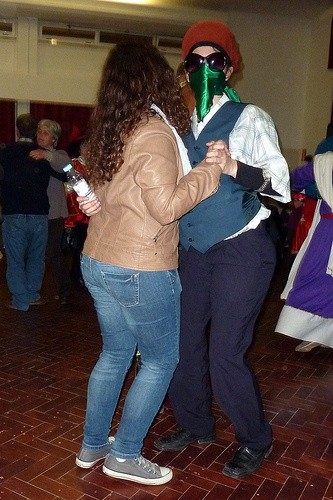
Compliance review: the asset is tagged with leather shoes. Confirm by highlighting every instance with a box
[153,429,217,452]
[222,437,273,478]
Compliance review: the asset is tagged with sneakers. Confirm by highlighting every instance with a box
[75,436,116,468]
[102,453,173,486]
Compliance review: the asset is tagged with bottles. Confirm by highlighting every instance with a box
[63,163,100,212]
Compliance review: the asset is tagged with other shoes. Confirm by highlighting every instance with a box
[294,340,321,352]
[4,300,29,311]
[60,295,71,305]
[29,297,50,305]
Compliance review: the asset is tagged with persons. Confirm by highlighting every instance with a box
[256,102,333,351]
[63,122,91,286]
[0,113,71,312]
[76,18,294,480]
[74,35,222,486]
[28,118,75,303]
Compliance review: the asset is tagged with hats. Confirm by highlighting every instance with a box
[181,20,241,74]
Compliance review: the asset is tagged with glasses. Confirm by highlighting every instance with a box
[184,52,227,73]
